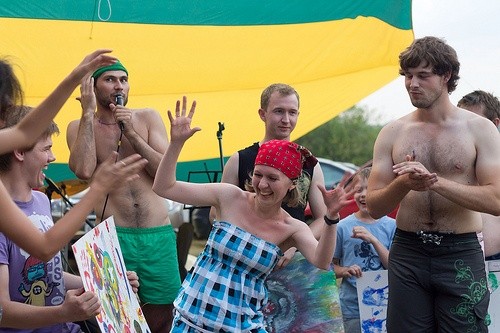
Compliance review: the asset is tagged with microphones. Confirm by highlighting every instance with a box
[115,93,124,131]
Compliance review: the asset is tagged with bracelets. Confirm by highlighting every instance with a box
[323,215,340,226]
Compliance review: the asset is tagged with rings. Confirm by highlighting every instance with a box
[119,161,126,166]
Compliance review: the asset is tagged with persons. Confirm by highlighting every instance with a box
[0,49,182,333]
[331,169,397,333]
[366,36,500,333]
[208,84,325,269]
[150,97,361,333]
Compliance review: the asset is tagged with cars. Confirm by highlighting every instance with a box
[304,157,400,220]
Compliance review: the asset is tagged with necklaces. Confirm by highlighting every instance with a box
[95,113,117,128]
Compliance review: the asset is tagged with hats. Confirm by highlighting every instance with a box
[92,58,128,80]
